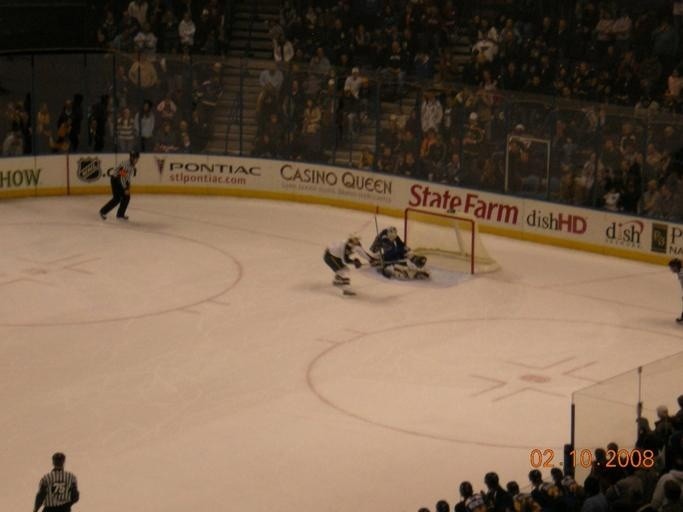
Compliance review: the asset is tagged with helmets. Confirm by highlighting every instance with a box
[386,227,398,241]
[52,453,65,466]
[668,258,680,273]
[419,468,563,512]
[130,149,140,161]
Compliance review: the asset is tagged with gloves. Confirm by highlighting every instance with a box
[353,258,361,268]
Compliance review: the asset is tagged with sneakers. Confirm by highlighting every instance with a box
[99,210,106,220]
[116,215,128,220]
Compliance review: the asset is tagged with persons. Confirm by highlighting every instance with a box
[0,0,233,159]
[369,227,412,268]
[31,452,79,512]
[98,149,141,220]
[380,252,431,281]
[248,0,681,227]
[418,393,682,512]
[323,231,380,297]
[668,257,682,323]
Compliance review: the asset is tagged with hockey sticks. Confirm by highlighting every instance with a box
[361,258,413,270]
[375,215,391,278]
